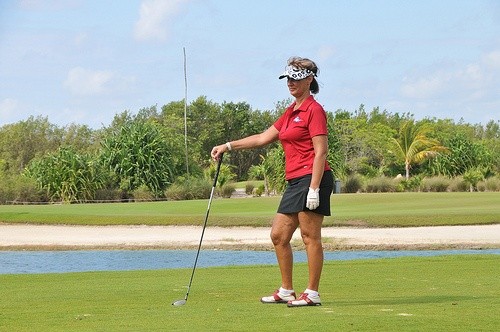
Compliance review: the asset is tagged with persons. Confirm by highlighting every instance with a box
[210,57,332,307]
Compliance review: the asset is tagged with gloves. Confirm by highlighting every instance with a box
[306,187,320,210]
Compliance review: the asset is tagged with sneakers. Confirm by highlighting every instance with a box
[261,289,297,303]
[287,292,322,307]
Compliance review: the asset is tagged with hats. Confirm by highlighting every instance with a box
[278,65,317,81]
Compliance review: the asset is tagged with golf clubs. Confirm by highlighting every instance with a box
[171,153,222,307]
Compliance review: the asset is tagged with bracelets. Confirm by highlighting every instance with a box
[226,142,232,151]
